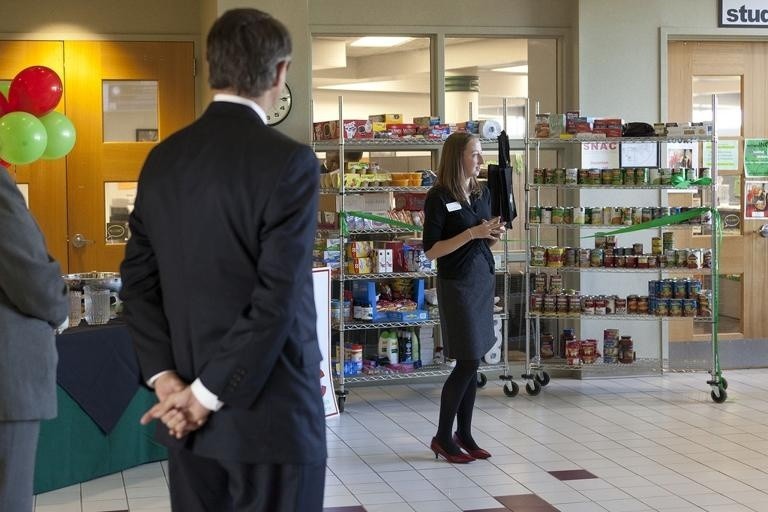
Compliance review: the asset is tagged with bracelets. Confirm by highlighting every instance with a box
[467,226,475,241]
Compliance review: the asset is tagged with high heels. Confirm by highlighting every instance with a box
[453,431,491,459]
[431,436,476,463]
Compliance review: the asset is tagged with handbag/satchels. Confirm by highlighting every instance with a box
[488,164,517,222]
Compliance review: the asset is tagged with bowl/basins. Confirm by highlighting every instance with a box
[61,272,121,295]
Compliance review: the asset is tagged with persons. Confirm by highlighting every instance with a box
[118,6,328,512]
[0,167,73,512]
[421,130,510,464]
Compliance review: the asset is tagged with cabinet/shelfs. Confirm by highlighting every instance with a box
[308,94,730,412]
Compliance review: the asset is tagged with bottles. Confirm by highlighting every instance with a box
[330,298,373,323]
[336,342,363,371]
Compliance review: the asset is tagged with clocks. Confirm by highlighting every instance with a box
[264,82,293,127]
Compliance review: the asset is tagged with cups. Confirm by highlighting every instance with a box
[65,278,121,329]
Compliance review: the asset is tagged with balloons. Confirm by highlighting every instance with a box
[0,65,78,168]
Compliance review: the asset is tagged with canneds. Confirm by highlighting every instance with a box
[540,332,555,359]
[529,205,712,225]
[530,272,626,317]
[626,277,713,318]
[529,112,711,141]
[559,328,597,365]
[534,166,709,187]
[531,231,712,270]
[602,329,633,364]
